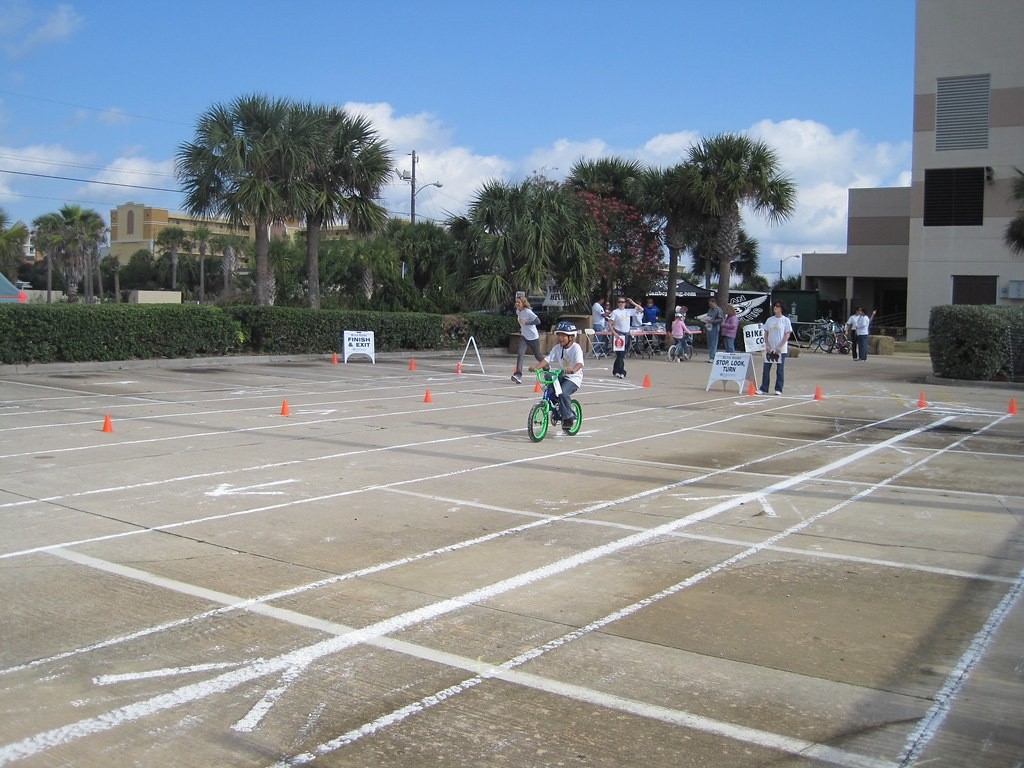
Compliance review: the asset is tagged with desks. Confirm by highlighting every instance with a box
[597,330,702,359]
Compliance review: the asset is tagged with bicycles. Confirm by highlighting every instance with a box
[797,319,852,354]
[667,332,693,362]
[528,368,582,443]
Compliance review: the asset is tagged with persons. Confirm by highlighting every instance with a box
[511,296,551,384]
[592,297,612,356]
[529,320,585,429]
[756,299,793,395]
[846,305,876,362]
[705,298,724,363]
[720,305,738,353]
[671,312,691,360]
[632,298,660,350]
[609,296,644,379]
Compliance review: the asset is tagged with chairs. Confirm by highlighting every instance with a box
[583,329,607,360]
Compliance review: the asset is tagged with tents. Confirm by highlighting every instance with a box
[645,277,717,298]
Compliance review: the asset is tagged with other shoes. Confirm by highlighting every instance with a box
[775,390,782,395]
[754,389,768,395]
[614,373,625,379]
[511,373,521,384]
[684,354,688,360]
[562,417,573,428]
[705,358,714,362]
[853,358,858,361]
[858,359,867,362]
[680,358,684,361]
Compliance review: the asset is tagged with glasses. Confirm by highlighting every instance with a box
[774,305,781,308]
[617,301,625,303]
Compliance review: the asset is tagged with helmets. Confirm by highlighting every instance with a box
[553,321,577,339]
[675,312,683,320]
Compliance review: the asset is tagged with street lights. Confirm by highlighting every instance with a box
[779,255,800,283]
[411,182,443,224]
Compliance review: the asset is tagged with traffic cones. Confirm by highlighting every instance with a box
[102,413,113,432]
[747,379,755,395]
[409,356,416,370]
[1007,396,1018,414]
[534,380,542,392]
[331,352,339,364]
[456,361,463,374]
[281,400,290,415]
[643,372,651,387]
[813,385,823,400]
[918,392,927,407]
[424,389,432,402]
